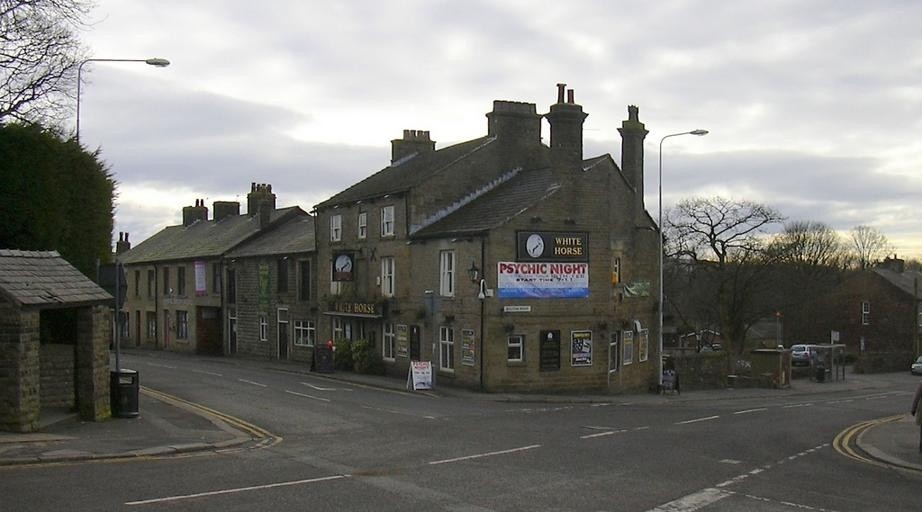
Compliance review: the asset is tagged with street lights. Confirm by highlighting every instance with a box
[72,52,171,149]
[657,128,710,395]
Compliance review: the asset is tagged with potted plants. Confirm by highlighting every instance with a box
[350,339,377,374]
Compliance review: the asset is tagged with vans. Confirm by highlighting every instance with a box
[789,342,821,366]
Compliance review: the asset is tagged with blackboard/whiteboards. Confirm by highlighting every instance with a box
[405,359,434,393]
[313,344,334,374]
[662,370,677,390]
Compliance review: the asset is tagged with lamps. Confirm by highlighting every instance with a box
[478,277,493,301]
[467,260,479,284]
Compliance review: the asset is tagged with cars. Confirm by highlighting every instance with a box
[911,355,922,376]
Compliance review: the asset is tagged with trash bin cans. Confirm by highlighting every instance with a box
[110,368,139,418]
[815,368,825,383]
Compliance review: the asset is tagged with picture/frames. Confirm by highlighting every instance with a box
[570,330,593,367]
[623,331,634,366]
[639,329,650,362]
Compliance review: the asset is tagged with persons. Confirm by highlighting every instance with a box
[810,350,819,369]
[911,382,922,453]
